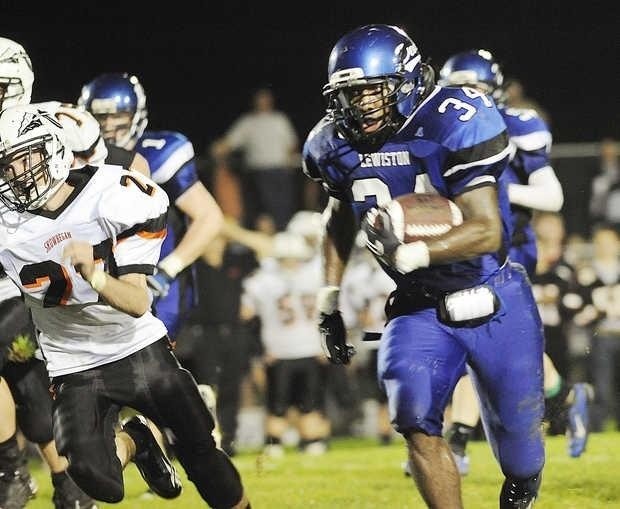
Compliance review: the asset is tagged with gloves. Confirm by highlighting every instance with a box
[358,215,430,275]
[146,257,184,318]
[317,286,356,365]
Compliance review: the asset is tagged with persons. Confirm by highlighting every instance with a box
[169,139,620,456]
[208,84,300,235]
[1,37,252,509]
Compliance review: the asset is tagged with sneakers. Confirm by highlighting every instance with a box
[0,469,37,509]
[198,384,221,448]
[404,451,469,478]
[563,382,595,457]
[121,414,183,500]
[52,476,98,509]
[500,472,542,509]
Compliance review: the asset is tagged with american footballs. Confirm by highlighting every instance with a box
[372,193,466,246]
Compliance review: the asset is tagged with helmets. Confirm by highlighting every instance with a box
[439,48,503,88]
[321,24,423,118]
[78,72,148,136]
[0,37,108,180]
